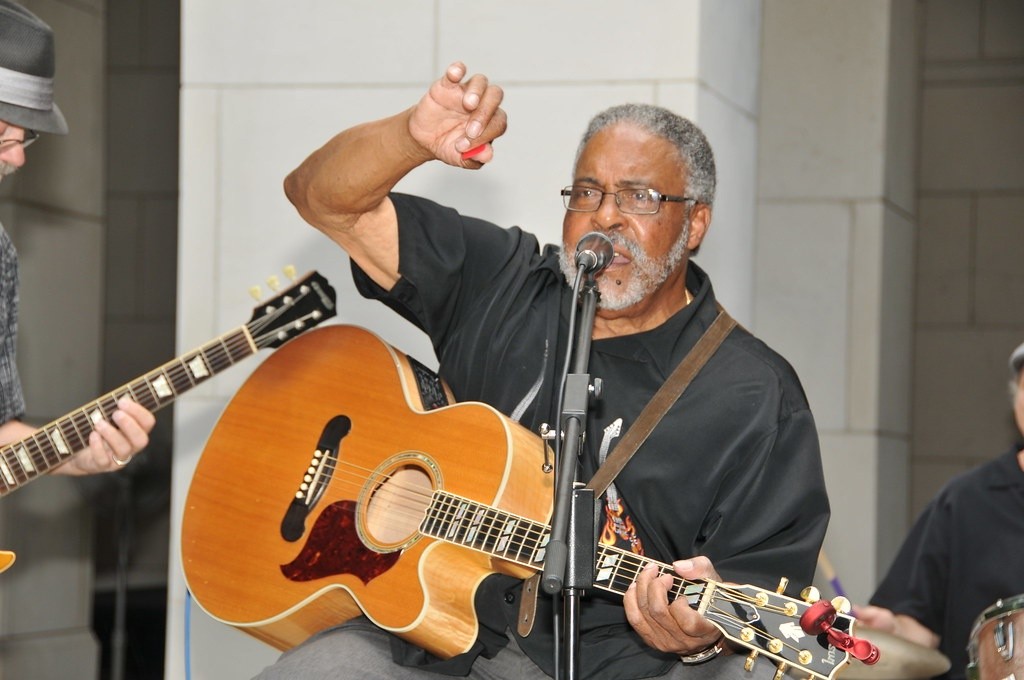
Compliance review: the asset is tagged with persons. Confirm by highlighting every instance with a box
[0,0,152,485]
[868,341,1023,680]
[246,60,832,680]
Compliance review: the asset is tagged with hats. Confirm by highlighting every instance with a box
[0,3,69,135]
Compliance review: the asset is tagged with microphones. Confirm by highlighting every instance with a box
[573,232,614,275]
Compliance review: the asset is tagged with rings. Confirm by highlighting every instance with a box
[111,453,131,466]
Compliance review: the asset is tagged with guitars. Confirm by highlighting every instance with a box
[180,323,880,680]
[1,271,338,573]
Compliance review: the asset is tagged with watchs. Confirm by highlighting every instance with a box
[682,635,724,666]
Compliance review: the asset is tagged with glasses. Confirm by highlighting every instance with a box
[561,185,690,215]
[0,130,38,150]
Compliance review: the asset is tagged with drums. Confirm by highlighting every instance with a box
[958,593,1024,680]
